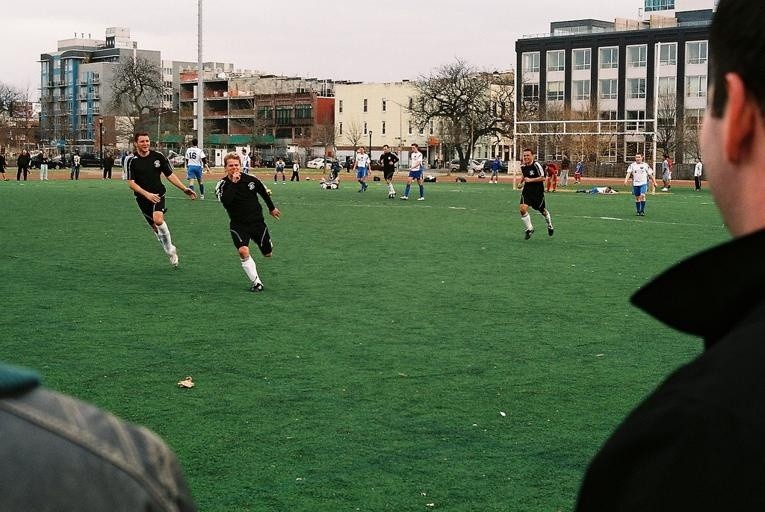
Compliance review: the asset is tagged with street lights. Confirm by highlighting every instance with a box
[156,110,177,147]
[382,98,403,169]
[368,131,373,160]
[97,116,104,170]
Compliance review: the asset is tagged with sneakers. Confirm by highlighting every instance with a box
[525,224,554,240]
[251,283,264,292]
[400,196,424,200]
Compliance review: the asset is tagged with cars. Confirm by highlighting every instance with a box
[5,148,187,170]
[446,159,508,173]
[247,153,398,172]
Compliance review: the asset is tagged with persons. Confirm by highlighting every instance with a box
[693,157,703,192]
[478,169,486,178]
[624,152,659,216]
[16,148,114,181]
[517,149,554,240]
[488,157,501,184]
[185,140,211,200]
[575,185,618,194]
[574,158,583,184]
[241,150,262,172]
[331,143,424,200]
[0,155,9,181]
[666,156,673,188]
[123,134,198,268]
[538,151,570,193]
[0,364,197,512]
[660,154,670,191]
[274,158,299,184]
[571,0,764,510]
[215,154,282,294]
